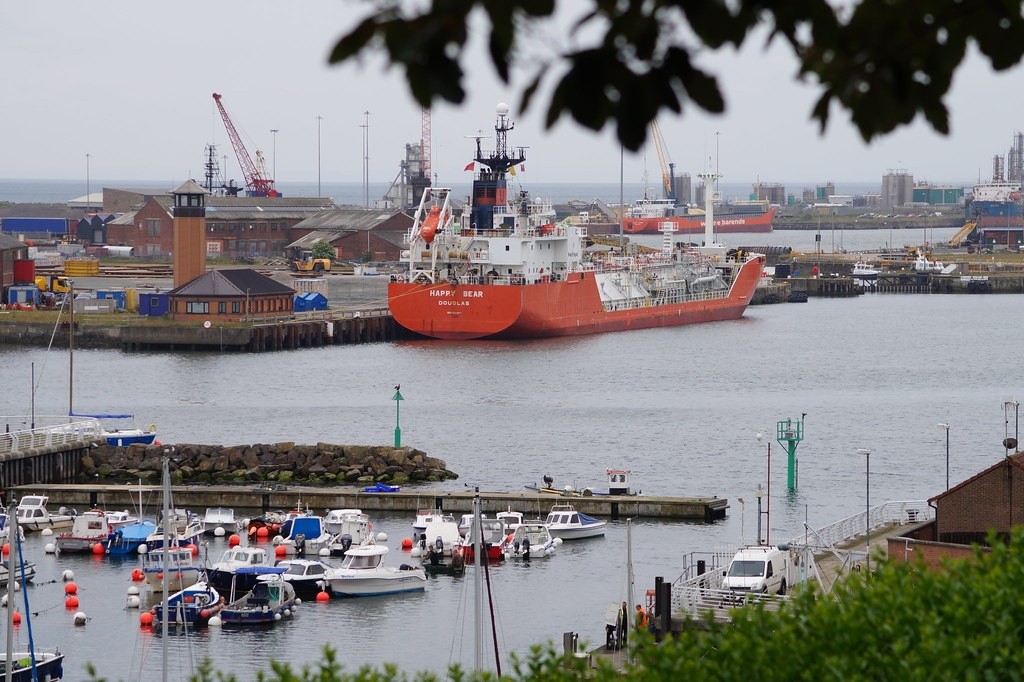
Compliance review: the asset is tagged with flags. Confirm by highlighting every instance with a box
[508,164,525,177]
[464,162,474,171]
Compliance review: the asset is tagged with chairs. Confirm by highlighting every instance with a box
[247,584,271,609]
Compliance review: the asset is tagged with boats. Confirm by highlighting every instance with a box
[525,469,645,497]
[322,533,427,599]
[155,572,219,626]
[619,199,775,235]
[0,644,64,682]
[421,560,463,573]
[0,478,155,588]
[137,508,372,595]
[388,102,767,340]
[220,568,296,625]
[410,500,606,560]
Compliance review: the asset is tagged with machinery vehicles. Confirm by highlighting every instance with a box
[290,251,331,279]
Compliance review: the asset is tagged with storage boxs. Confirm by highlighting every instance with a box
[366,482,403,492]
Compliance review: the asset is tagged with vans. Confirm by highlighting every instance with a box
[721,544,787,600]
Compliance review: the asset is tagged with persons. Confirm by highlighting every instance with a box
[446,550,464,568]
[422,545,440,566]
[617,601,650,649]
[390,267,561,289]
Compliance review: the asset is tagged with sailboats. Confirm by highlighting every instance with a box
[9,282,157,445]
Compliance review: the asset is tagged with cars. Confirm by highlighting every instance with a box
[75,293,92,300]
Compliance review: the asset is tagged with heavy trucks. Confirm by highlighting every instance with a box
[579,199,617,224]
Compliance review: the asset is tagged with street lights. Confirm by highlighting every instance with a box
[937,422,949,490]
[1010,400,1019,453]
[857,448,870,551]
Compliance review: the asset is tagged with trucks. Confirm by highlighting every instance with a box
[35,276,71,295]
